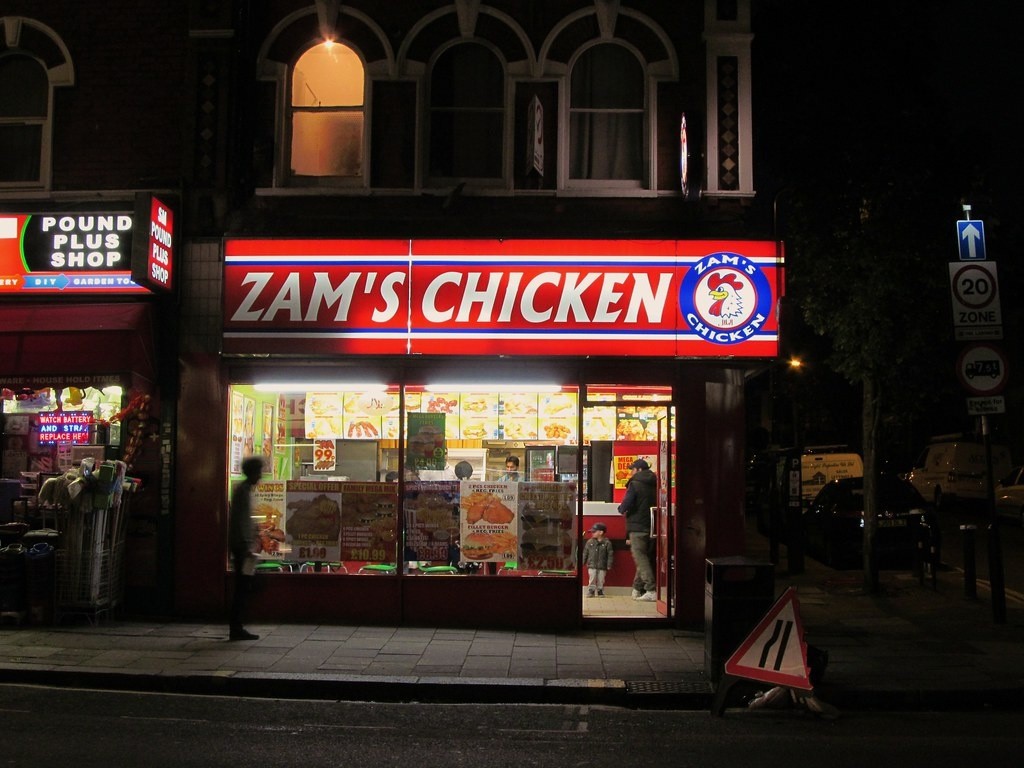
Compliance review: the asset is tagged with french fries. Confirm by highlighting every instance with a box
[425,443,433,451]
[434,434,443,441]
[416,508,459,539]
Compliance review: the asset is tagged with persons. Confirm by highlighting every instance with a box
[495,456,525,482]
[228,458,270,641]
[617,459,658,601]
[582,522,613,598]
[385,471,398,483]
[454,461,474,481]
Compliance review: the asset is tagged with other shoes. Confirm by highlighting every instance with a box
[631,591,641,600]
[587,590,595,597]
[229,630,259,640]
[598,590,604,597]
[637,592,656,601]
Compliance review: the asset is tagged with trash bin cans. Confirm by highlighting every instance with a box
[23,536,68,625]
[0,521,29,626]
[704,556,775,682]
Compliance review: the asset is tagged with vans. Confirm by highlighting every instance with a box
[768,444,861,512]
[902,433,1012,508]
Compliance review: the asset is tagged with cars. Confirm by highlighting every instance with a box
[803,473,943,567]
[994,464,1024,520]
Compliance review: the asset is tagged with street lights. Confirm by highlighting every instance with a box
[784,353,809,446]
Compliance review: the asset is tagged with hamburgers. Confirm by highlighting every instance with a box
[521,500,572,532]
[520,526,571,569]
[409,425,441,456]
[359,498,394,527]
[462,532,494,560]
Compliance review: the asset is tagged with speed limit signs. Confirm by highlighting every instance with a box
[947,260,1003,342]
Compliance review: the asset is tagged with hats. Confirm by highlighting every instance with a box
[588,522,605,532]
[628,459,648,468]
